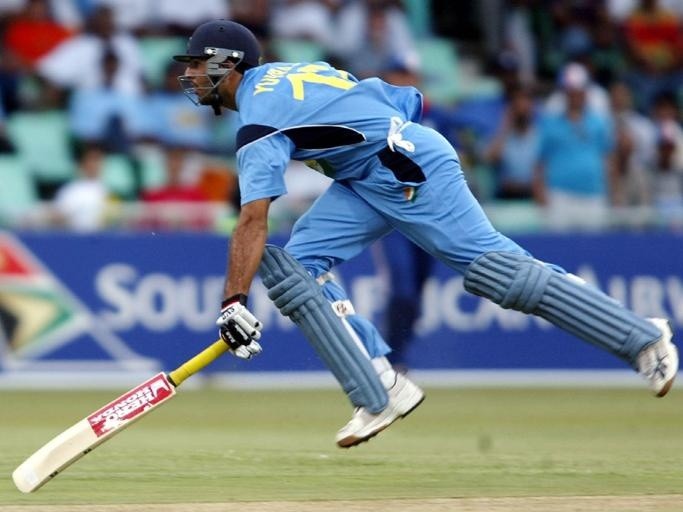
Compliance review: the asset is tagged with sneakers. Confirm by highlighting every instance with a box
[335,373,424,446]
[636,318,678,398]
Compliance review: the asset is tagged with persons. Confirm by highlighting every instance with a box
[173,18,679,448]
[0,1,681,233]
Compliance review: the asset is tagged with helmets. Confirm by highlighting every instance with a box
[172,19,260,106]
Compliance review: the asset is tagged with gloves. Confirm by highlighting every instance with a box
[217,294,262,360]
